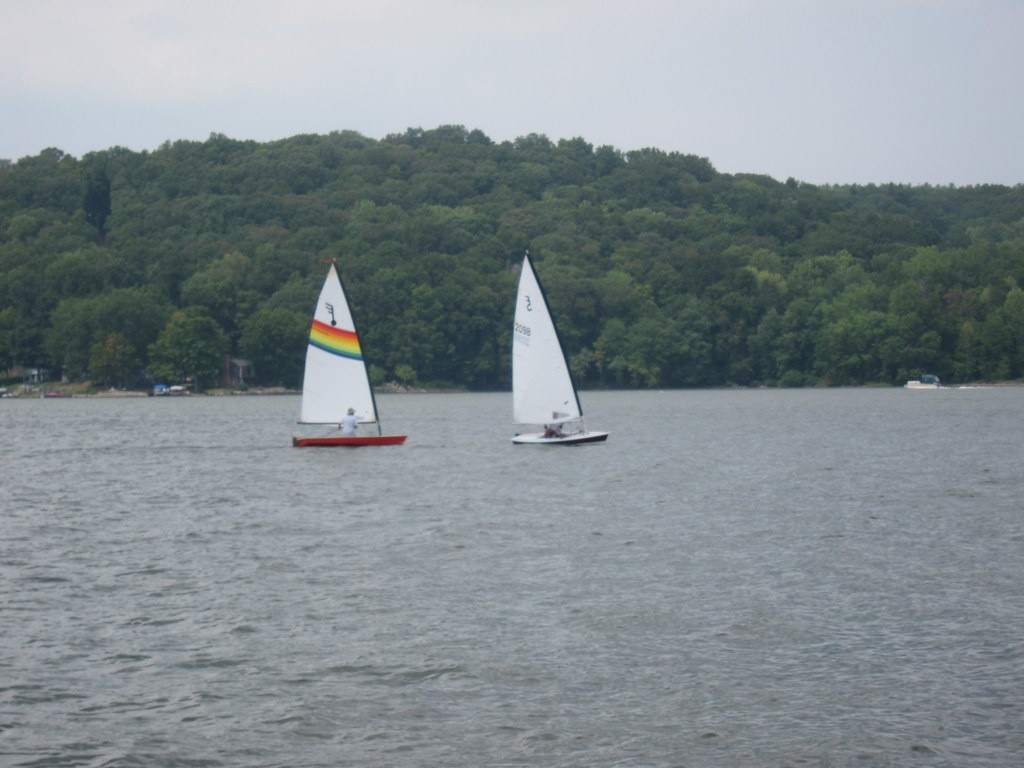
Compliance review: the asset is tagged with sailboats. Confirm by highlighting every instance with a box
[293,258,409,447]
[511,250,610,444]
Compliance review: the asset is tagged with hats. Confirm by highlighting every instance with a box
[347,408,355,414]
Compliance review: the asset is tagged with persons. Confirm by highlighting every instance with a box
[337,409,358,437]
[545,412,566,438]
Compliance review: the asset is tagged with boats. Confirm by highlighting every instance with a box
[903,380,936,389]
[164,385,188,396]
